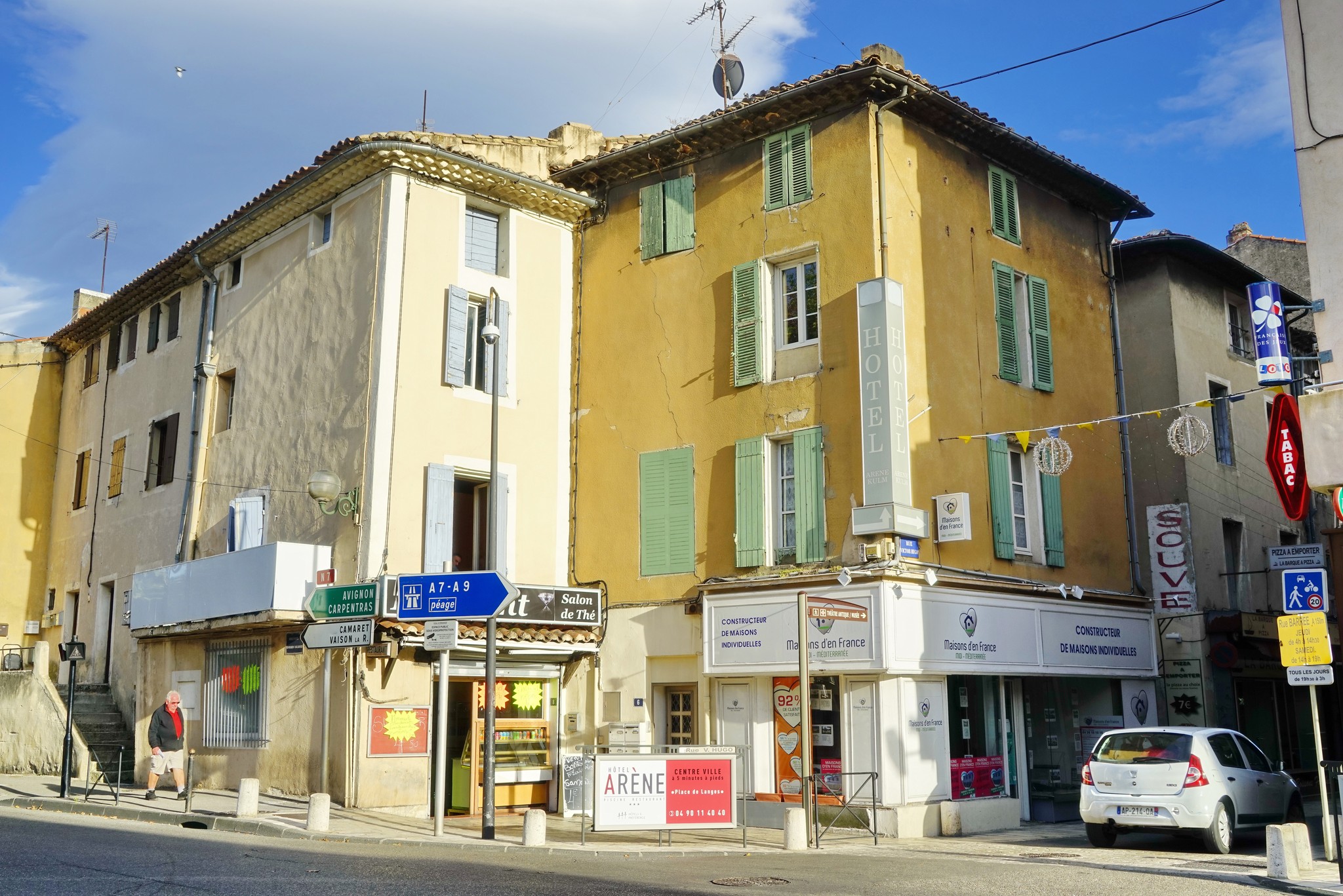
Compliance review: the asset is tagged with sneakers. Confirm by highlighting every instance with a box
[177,788,195,800]
[146,790,158,801]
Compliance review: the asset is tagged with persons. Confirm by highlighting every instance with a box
[145,690,195,801]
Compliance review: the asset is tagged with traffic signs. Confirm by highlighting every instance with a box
[395,570,521,620]
[810,608,871,622]
[300,619,374,649]
[302,582,380,622]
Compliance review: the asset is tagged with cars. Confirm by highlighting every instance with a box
[1079,726,1307,855]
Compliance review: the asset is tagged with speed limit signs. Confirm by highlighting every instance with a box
[1308,594,1322,609]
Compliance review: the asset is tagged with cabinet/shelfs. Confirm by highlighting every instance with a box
[461,727,552,773]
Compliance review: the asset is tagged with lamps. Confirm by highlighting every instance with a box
[1033,584,1084,600]
[837,566,871,587]
[895,568,938,586]
[1166,633,1182,642]
[308,470,359,524]
[1151,595,1180,608]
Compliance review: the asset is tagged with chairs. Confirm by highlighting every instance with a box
[1,645,23,671]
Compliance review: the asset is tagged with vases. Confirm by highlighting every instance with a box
[755,792,844,805]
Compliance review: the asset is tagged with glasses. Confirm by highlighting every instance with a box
[168,700,180,704]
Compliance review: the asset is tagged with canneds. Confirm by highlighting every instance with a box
[495,731,530,740]
[480,730,484,740]
[530,729,543,739]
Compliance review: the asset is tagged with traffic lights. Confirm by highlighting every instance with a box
[64,641,86,662]
